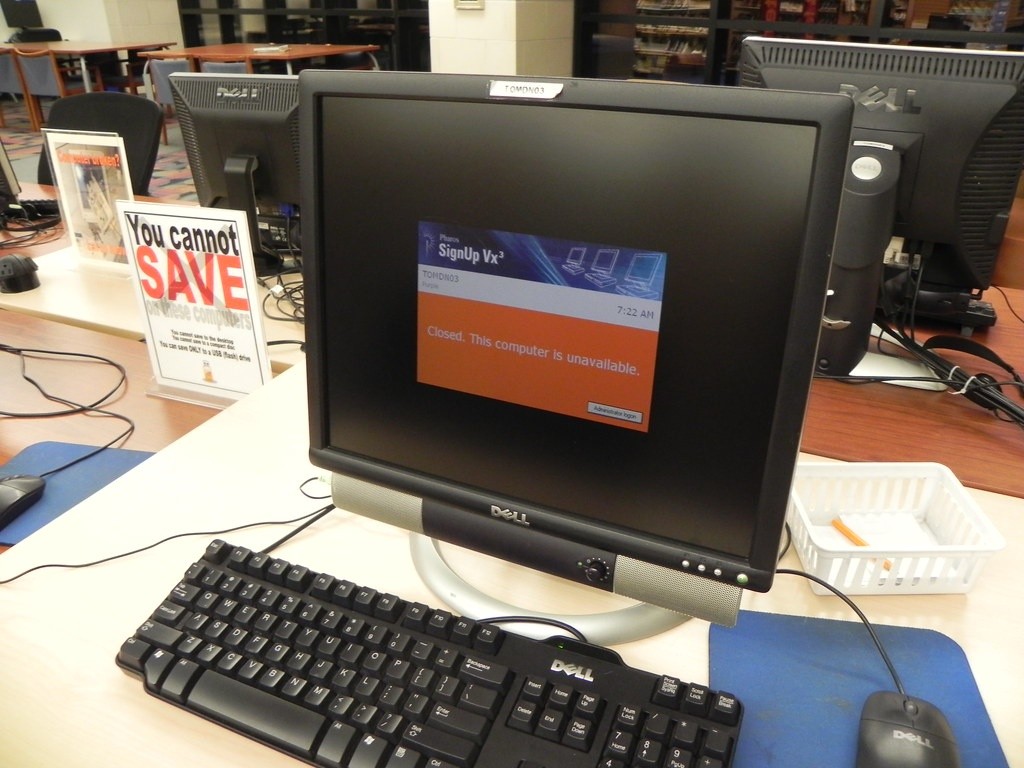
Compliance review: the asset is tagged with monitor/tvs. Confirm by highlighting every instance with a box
[0,138,22,227]
[166,36,1024,644]
[0,0,43,41]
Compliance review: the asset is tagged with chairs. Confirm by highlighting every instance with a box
[38,92,164,194]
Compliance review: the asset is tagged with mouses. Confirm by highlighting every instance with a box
[853,689,961,768]
[0,473,45,530]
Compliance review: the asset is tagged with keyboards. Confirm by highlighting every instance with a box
[964,298,998,327]
[19,200,60,216]
[113,539,743,768]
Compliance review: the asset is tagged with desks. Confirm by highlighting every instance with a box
[0,39,1024,768]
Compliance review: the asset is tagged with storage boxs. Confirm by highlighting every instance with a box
[786,461,1003,597]
